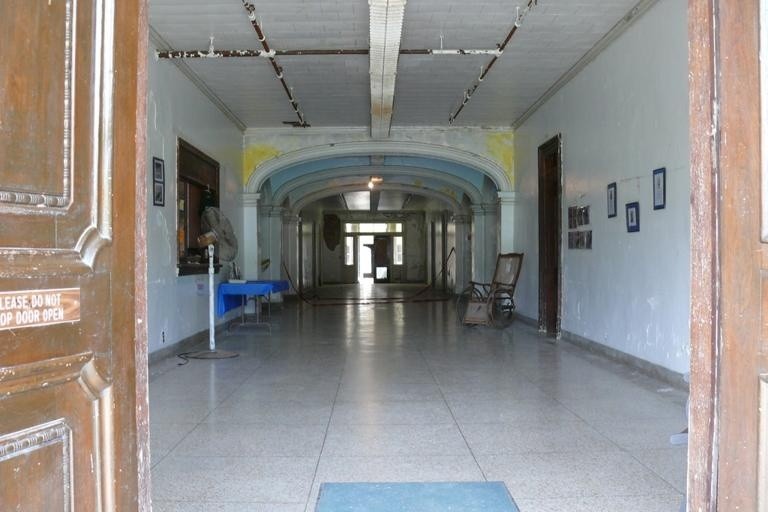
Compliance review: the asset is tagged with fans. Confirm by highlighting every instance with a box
[185,206,239,359]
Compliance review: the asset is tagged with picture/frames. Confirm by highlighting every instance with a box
[153,155,166,207]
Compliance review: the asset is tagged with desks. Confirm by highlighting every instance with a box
[220,281,289,335]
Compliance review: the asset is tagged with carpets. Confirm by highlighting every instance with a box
[312,481,521,512]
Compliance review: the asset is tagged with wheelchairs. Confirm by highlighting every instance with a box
[456,250,524,330]
